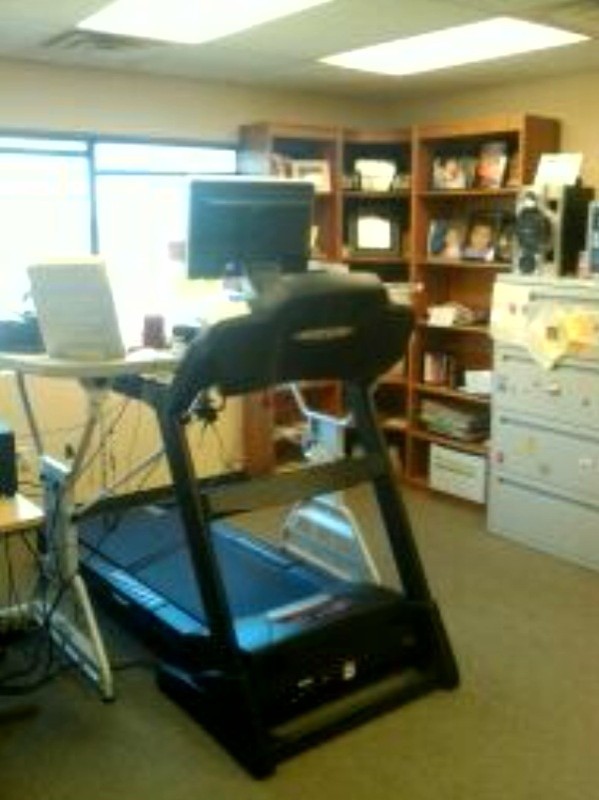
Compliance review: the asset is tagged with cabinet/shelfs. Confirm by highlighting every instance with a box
[487,273,598,572]
[234,112,558,504]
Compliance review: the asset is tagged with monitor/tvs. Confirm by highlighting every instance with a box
[30,262,127,359]
[187,178,313,278]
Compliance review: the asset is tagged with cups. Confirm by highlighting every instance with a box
[423,351,446,385]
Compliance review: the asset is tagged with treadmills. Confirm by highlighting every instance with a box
[34,172,463,781]
